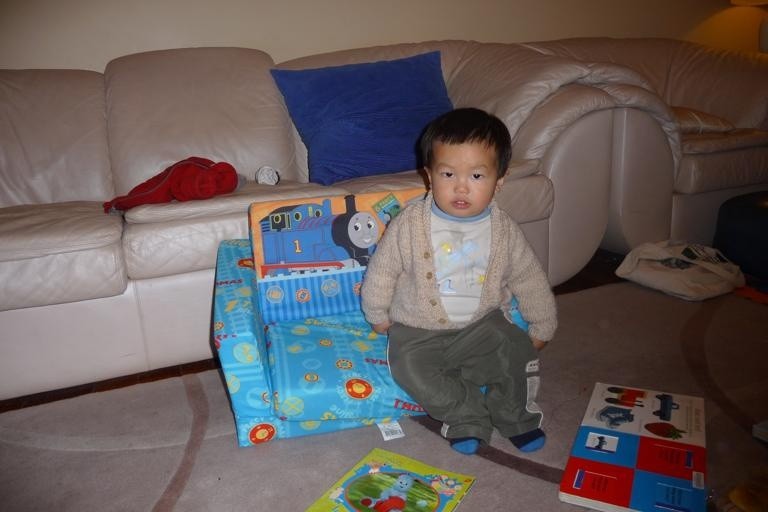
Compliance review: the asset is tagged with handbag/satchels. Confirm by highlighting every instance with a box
[614,238,748,303]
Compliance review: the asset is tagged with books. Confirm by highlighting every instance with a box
[306,448,474,512]
[559,383,709,512]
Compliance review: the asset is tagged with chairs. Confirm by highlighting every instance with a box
[515,38,768,255]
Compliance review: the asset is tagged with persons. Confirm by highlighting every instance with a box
[361,108,559,455]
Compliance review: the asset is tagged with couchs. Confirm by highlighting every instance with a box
[0,41,616,400]
[213,187,530,447]
